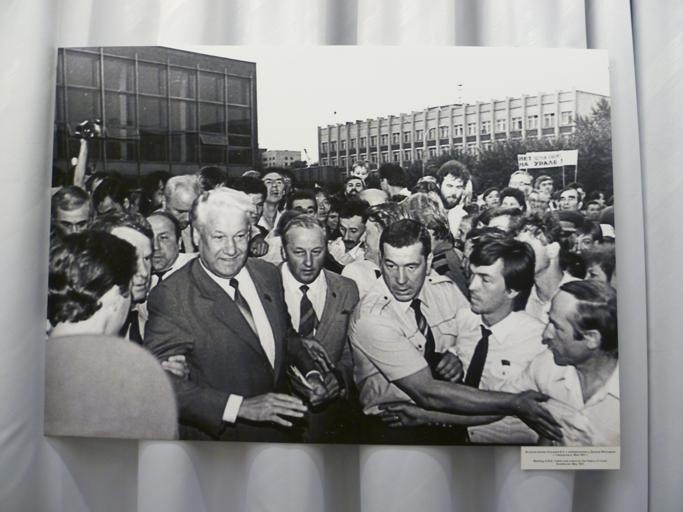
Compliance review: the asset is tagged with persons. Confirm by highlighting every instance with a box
[44,121,621,449]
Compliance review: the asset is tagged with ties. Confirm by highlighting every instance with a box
[129,310,143,345]
[409,299,435,361]
[299,286,319,338]
[465,324,491,387]
[152,268,173,287]
[229,278,259,343]
